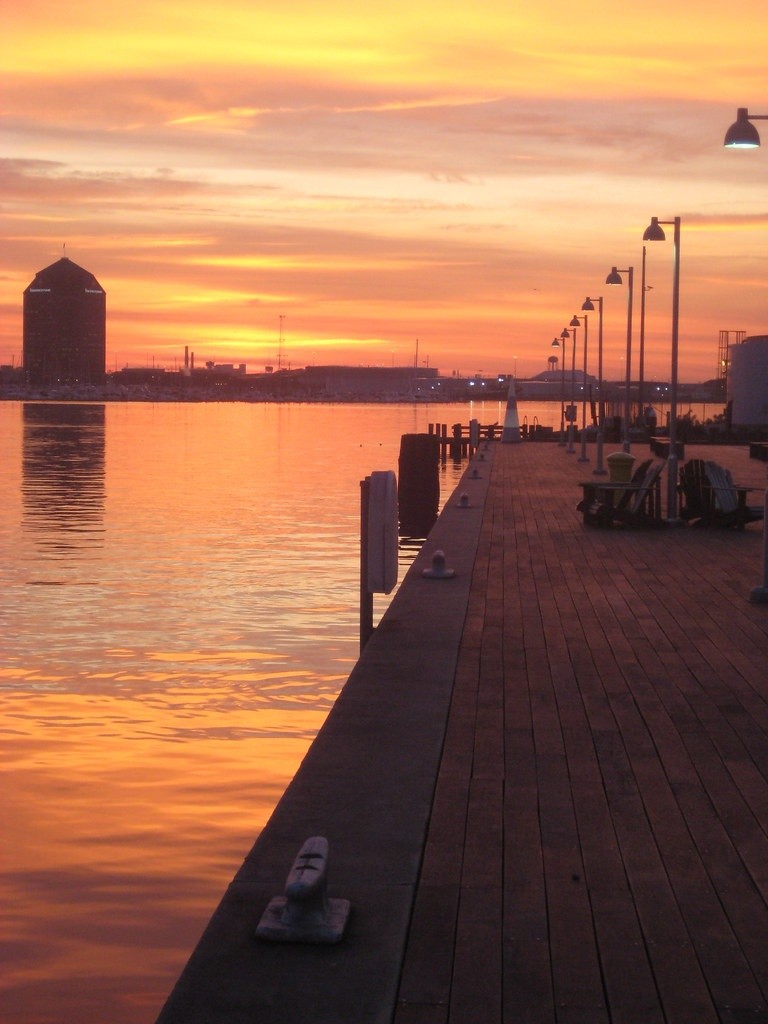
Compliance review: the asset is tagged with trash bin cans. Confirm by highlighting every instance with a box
[608,453,637,510]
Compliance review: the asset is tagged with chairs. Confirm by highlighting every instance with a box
[577,458,665,529]
[677,459,766,533]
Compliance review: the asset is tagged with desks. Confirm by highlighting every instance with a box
[750,442,768,460]
[650,437,685,460]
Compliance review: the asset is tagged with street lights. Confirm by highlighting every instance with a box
[604,267,635,454]
[569,315,589,462]
[582,296,608,476]
[642,216,679,527]
[561,328,576,454]
[552,337,567,448]
